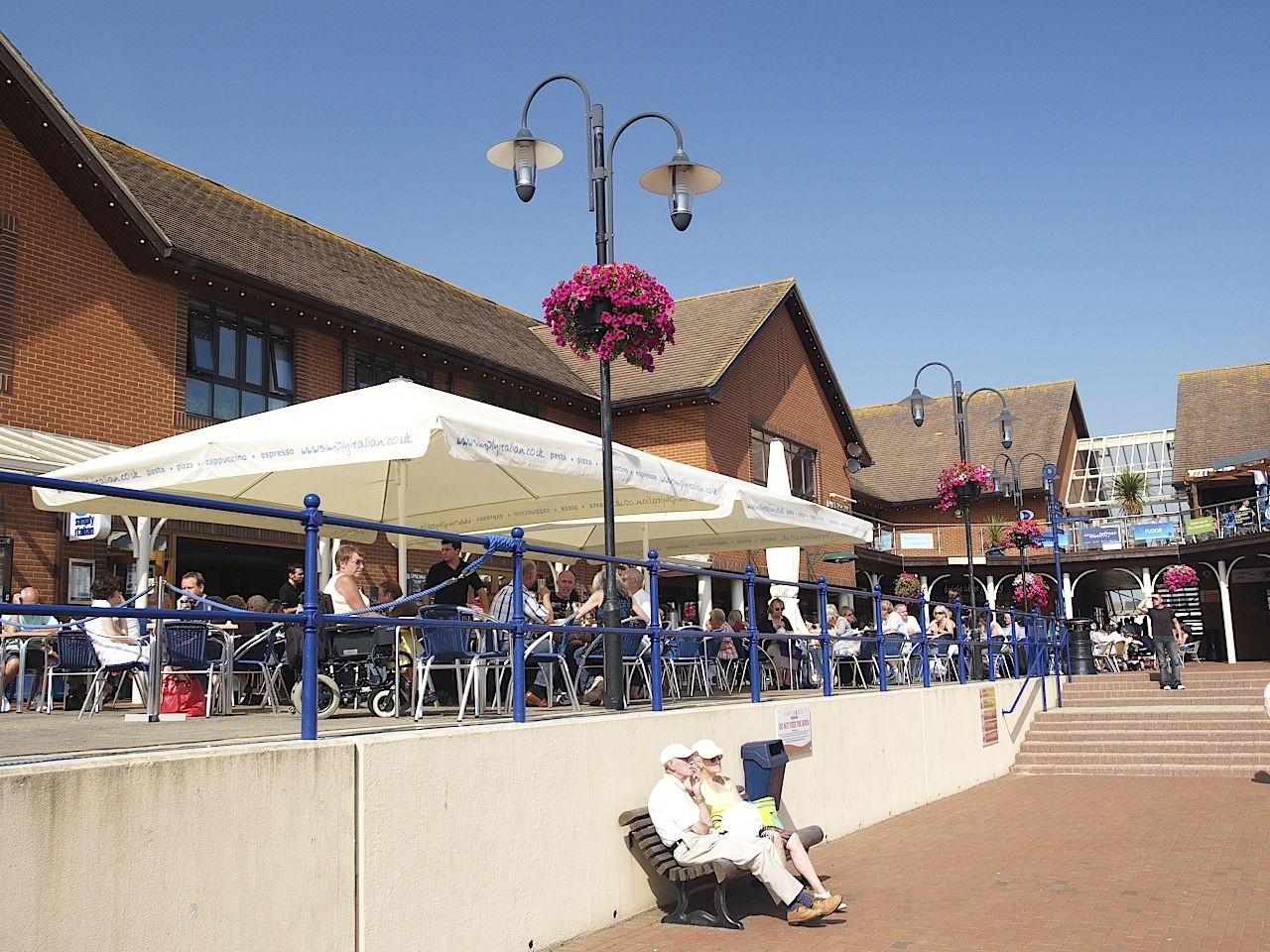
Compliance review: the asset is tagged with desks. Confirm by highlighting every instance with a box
[553,619,599,624]
[393,616,488,719]
[0,628,56,713]
[145,623,239,722]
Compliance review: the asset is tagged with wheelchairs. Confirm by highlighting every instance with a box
[291,591,439,720]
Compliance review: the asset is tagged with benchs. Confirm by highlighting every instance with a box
[618,785,824,930]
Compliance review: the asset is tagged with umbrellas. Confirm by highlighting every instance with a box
[29,377,726,599]
[388,448,876,594]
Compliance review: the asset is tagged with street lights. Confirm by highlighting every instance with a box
[894,362,1023,682]
[485,76,724,711]
[991,454,1048,612]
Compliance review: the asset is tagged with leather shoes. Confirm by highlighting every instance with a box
[525,691,548,707]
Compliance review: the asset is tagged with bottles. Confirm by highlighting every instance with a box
[669,602,678,630]
[565,602,577,617]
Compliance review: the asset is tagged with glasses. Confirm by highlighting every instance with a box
[705,755,723,762]
[845,612,854,616]
[348,560,364,565]
[934,612,945,615]
[771,607,785,611]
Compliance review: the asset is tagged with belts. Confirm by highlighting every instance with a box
[670,839,683,851]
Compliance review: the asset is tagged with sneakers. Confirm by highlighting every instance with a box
[810,894,842,921]
[31,693,47,712]
[552,690,572,705]
[814,891,847,910]
[786,904,825,926]
[0,696,11,713]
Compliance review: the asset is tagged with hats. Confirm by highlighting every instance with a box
[692,739,723,759]
[659,744,698,766]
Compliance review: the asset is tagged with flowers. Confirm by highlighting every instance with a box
[1012,572,1048,607]
[1163,564,1199,592]
[893,571,921,606]
[1000,518,1044,551]
[541,262,676,373]
[937,459,994,514]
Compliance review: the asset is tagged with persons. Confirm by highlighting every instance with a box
[648,743,844,926]
[1202,501,1260,542]
[1176,625,1195,656]
[0,562,303,712]
[1145,515,1168,548]
[1089,617,1156,671]
[1137,592,1186,690]
[319,538,1026,708]
[692,740,847,910]
[1263,681,1270,717]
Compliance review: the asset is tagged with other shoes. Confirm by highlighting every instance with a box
[773,666,905,690]
[583,675,604,703]
[102,693,114,706]
[1163,685,1170,690]
[409,687,441,707]
[1177,685,1186,690]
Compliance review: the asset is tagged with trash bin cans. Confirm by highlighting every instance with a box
[741,740,792,813]
[1065,618,1098,675]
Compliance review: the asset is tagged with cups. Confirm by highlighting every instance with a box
[537,579,546,602]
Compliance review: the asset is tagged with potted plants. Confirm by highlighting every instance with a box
[982,515,1007,557]
[1110,470,1146,516]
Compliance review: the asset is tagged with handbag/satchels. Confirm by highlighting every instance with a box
[160,664,206,717]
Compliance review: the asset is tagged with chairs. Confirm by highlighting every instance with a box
[1178,641,1202,668]
[1093,639,1156,673]
[0,603,1069,723]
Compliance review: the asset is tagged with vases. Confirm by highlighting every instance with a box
[573,297,612,336]
[954,482,979,505]
